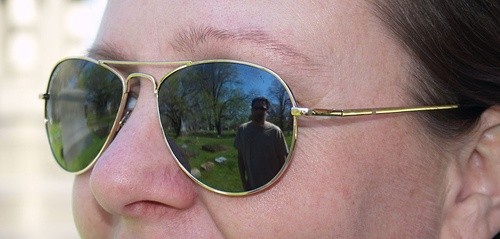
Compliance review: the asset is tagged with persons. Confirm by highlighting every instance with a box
[233,97,289,191]
[37,0,500,239]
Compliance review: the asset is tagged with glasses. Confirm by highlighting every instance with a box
[38,57,478,196]
